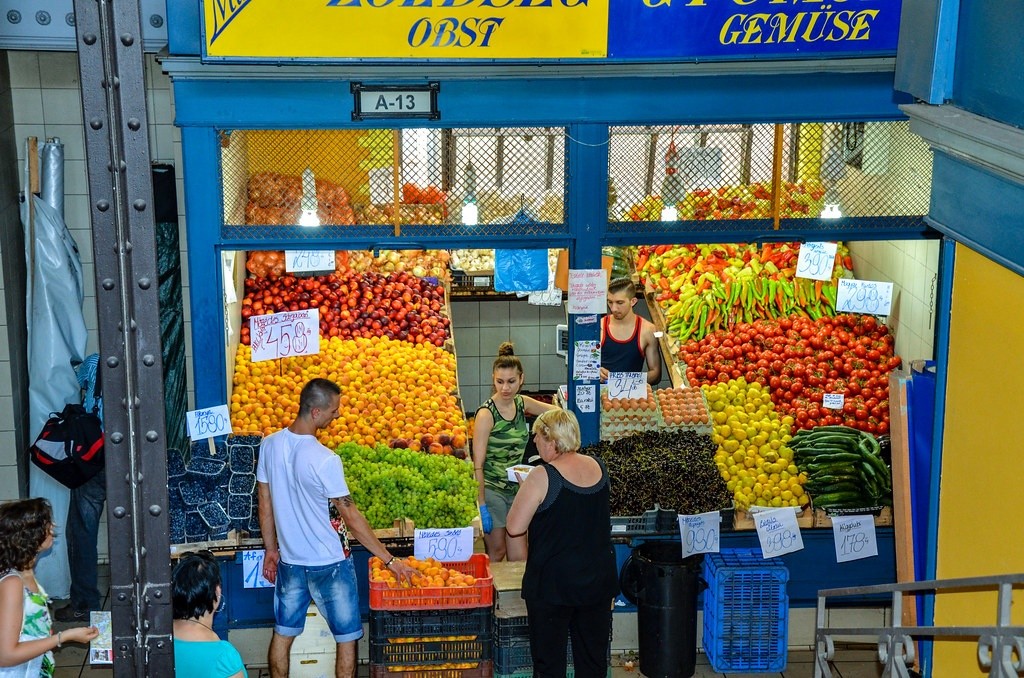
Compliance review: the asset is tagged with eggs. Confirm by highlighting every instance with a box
[599,382,710,440]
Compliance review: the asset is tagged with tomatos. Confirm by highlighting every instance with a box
[679,313,902,436]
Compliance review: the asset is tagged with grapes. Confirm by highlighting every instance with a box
[333,442,481,529]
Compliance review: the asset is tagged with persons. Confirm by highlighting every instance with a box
[171,553,248,678]
[255,378,421,678]
[0,497,98,677]
[472,342,566,563]
[54,353,107,623]
[505,410,620,678]
[600,277,663,385]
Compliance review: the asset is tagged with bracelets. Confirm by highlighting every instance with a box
[384,555,396,566]
[57,631,62,648]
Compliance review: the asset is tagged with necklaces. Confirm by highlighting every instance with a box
[181,617,214,632]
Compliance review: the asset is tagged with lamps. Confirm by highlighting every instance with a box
[660,125,680,221]
[821,124,843,218]
[462,129,480,227]
[299,131,321,226]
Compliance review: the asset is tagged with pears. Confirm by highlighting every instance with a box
[700,376,809,510]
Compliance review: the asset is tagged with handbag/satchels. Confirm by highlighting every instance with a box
[29,355,107,487]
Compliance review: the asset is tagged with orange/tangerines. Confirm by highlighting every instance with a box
[371,555,478,589]
[229,337,471,460]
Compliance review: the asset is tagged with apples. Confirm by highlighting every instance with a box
[241,268,451,348]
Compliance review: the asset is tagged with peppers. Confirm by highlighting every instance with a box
[621,181,856,340]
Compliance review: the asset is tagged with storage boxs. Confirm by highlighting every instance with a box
[448,261,494,292]
[493,609,613,647]
[609,505,658,535]
[368,639,492,664]
[495,667,612,678]
[368,552,494,611]
[656,501,734,534]
[494,645,611,675]
[369,662,493,678]
[369,608,495,639]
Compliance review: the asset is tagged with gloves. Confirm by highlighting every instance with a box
[479,504,493,535]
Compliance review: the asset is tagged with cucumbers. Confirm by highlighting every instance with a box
[786,426,894,515]
